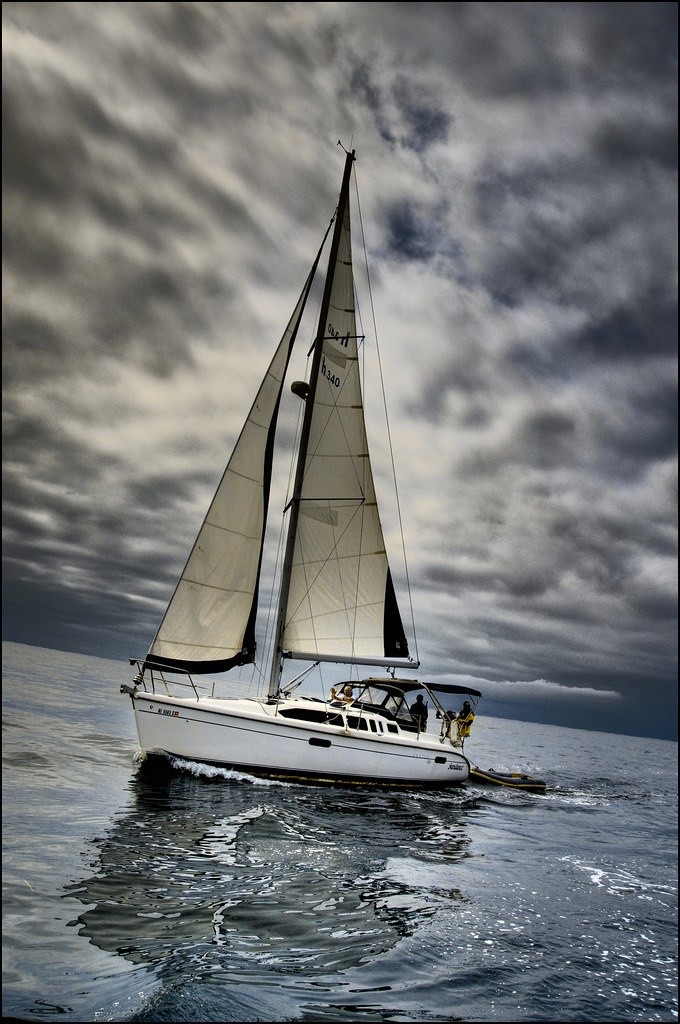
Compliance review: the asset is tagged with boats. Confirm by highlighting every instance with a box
[468,765,547,794]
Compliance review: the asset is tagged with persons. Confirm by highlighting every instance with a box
[388,698,409,720]
[330,686,353,703]
[409,695,428,723]
[457,701,475,735]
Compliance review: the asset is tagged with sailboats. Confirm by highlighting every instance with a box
[118,116,472,792]
